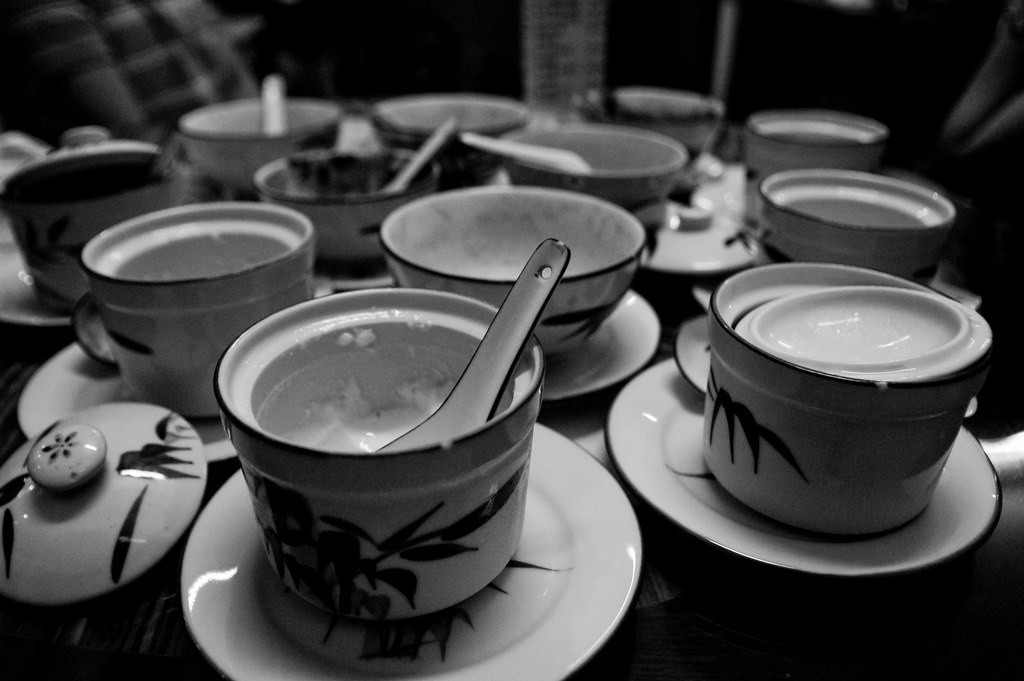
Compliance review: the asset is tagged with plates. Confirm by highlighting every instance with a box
[0,116,1001,681]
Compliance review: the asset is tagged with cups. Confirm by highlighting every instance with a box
[212,288,546,622]
[763,168,957,286]
[180,95,343,189]
[588,91,725,168]
[369,95,528,185]
[253,147,440,279]
[497,127,689,257]
[380,186,647,365]
[0,128,180,304]
[745,115,890,228]
[81,200,316,417]
[704,263,993,537]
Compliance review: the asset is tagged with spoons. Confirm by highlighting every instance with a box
[381,236,570,460]
